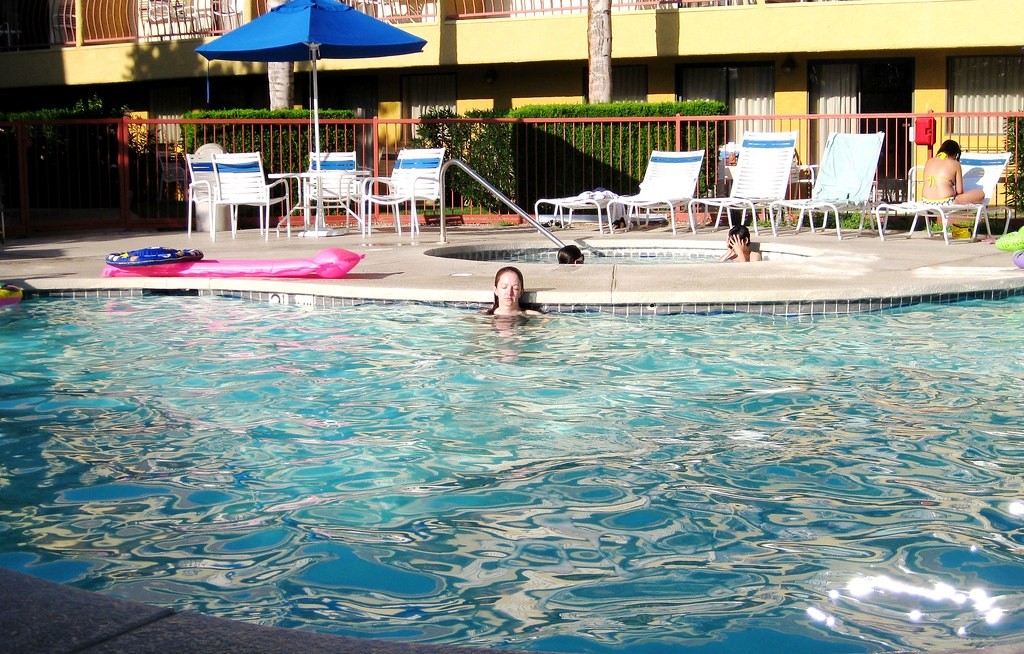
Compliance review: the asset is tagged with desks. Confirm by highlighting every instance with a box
[266,170,372,235]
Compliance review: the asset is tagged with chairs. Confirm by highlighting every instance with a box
[689,129,798,235]
[187,151,290,241]
[302,151,367,229]
[362,146,447,245]
[149,1,244,34]
[535,188,628,235]
[768,129,886,239]
[876,152,1014,241]
[606,148,707,236]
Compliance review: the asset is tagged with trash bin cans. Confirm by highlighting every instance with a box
[195,142,232,233]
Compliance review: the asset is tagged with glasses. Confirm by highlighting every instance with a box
[575,254,584,264]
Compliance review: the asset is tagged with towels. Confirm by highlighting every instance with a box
[577,186,633,227]
[805,132,884,204]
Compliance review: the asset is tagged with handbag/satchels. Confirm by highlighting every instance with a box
[951,223,972,239]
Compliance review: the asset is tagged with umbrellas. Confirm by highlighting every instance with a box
[194,0,429,226]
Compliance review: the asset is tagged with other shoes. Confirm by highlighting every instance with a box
[618,217,624,229]
[612,220,619,229]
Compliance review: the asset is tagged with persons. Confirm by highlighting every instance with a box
[924,139,985,207]
[558,244,587,265]
[714,225,764,263]
[481,267,542,315]
